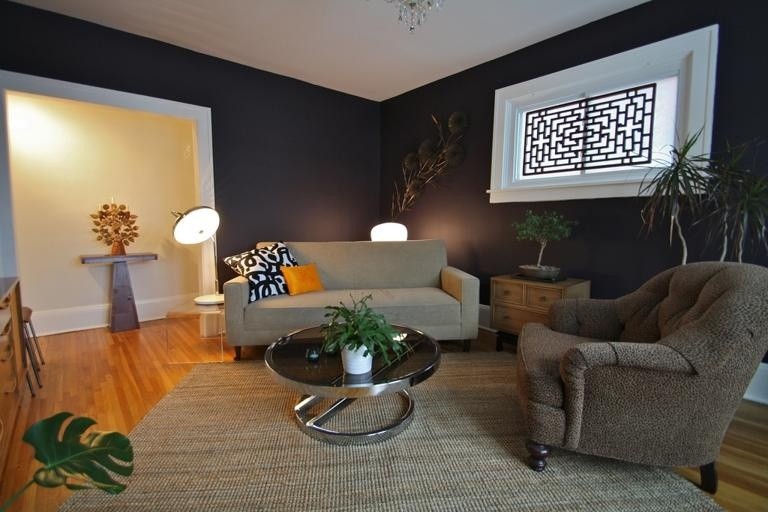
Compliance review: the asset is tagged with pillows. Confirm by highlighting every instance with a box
[223,240,299,303]
[280,262,323,296]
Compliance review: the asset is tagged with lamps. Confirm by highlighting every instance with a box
[170,206,224,305]
[386,0,441,34]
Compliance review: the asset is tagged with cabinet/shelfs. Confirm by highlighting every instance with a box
[1,281,28,481]
[489,274,591,351]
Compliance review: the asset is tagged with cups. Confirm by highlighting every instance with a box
[305,346,320,364]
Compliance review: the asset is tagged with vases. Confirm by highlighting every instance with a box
[112,232,126,255]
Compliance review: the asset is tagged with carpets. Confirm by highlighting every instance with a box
[57,349,725,512]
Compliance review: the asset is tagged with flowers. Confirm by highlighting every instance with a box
[89,203,140,245]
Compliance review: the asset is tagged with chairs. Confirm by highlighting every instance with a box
[21,304,45,399]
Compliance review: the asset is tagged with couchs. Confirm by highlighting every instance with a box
[223,239,480,360]
[518,261,768,494]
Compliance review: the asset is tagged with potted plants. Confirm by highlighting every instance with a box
[319,293,413,375]
[513,209,580,282]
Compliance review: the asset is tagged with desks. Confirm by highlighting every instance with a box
[167,297,225,363]
[81,253,157,333]
